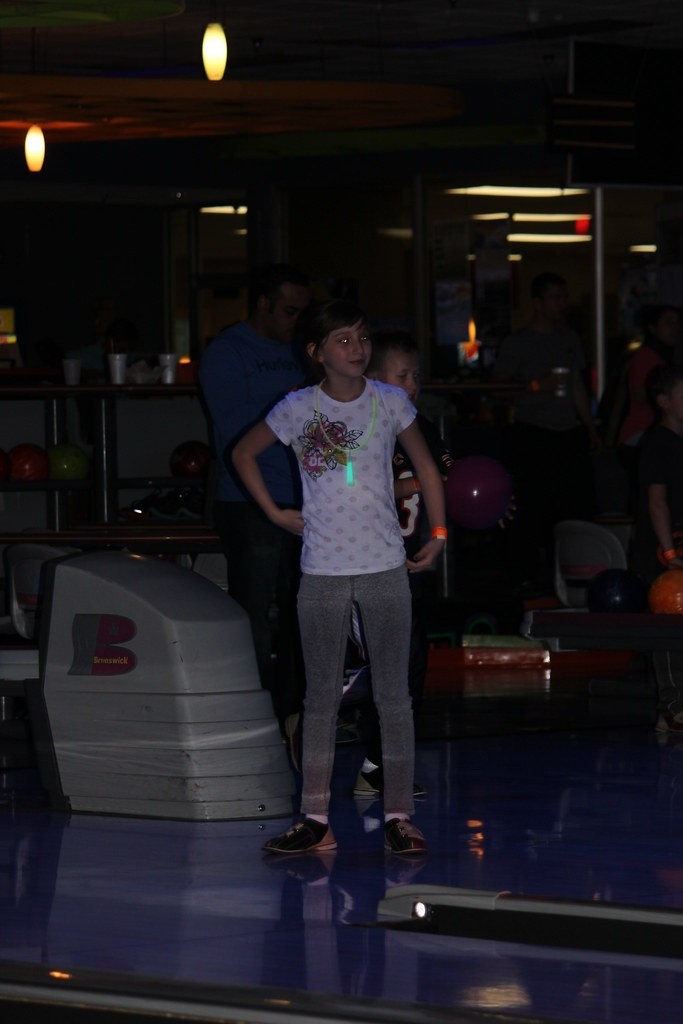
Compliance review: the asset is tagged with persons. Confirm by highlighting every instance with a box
[488,272,683,732]
[195,262,517,796]
[232,299,445,852]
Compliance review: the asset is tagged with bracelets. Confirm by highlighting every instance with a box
[429,525,448,540]
[413,475,421,491]
[663,548,677,560]
[529,378,542,394]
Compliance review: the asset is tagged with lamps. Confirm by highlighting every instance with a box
[202,0,227,81]
[24,120,46,173]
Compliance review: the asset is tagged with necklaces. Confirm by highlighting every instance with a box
[315,375,376,453]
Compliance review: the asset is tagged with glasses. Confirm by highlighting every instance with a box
[270,298,305,320]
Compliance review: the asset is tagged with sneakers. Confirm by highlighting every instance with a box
[261,851,336,884]
[261,818,339,854]
[384,818,429,855]
[354,795,427,816]
[351,766,430,797]
[385,852,425,884]
[296,712,358,742]
[658,733,683,749]
[285,712,302,774]
[656,711,683,734]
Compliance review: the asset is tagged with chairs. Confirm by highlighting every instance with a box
[553,517,629,608]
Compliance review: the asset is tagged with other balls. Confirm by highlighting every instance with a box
[446,456,513,530]
[46,444,88,481]
[1,447,10,482]
[587,568,648,615]
[647,568,683,615]
[170,441,213,482]
[7,444,51,480]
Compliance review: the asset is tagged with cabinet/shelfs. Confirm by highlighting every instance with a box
[0,383,219,556]
